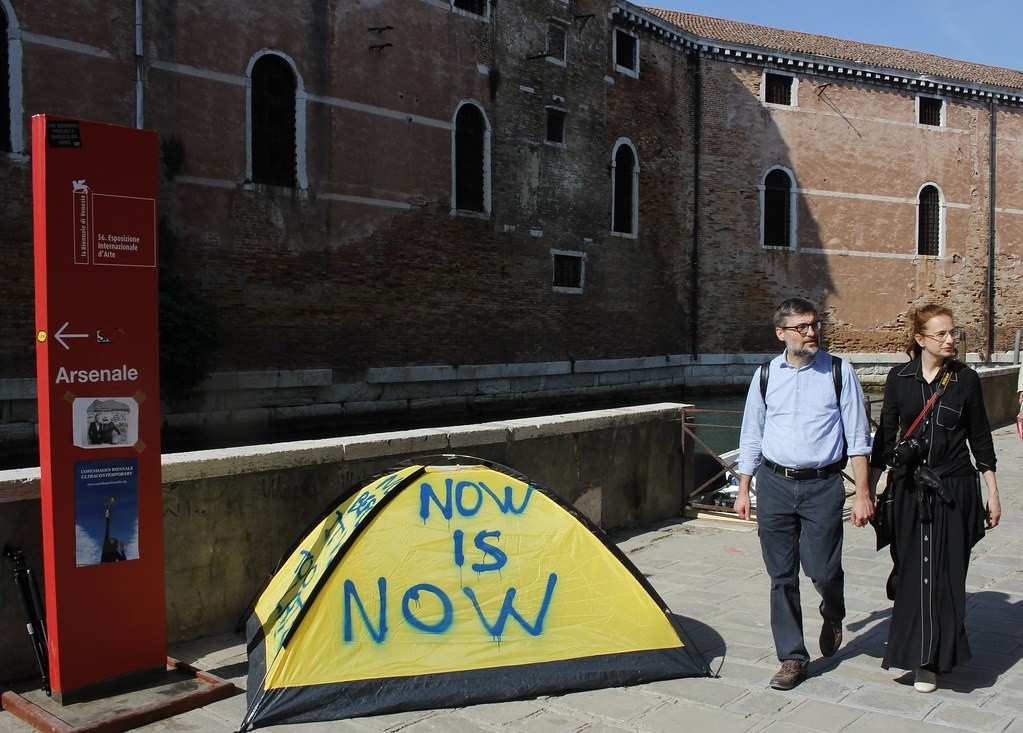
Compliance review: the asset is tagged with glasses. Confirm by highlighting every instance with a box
[783,319,823,334]
[922,329,961,341]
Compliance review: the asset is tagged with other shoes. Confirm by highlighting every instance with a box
[914,669,936,693]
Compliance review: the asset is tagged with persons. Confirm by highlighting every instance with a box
[868,304,1001,693]
[733,298,875,690]
[1016,357,1023,405]
[100,512,127,564]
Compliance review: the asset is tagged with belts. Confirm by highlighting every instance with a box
[760,456,842,481]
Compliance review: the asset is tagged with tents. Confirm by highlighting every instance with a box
[234,453,721,733]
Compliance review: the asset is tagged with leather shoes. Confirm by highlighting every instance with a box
[769,658,807,689]
[820,619,843,657]
